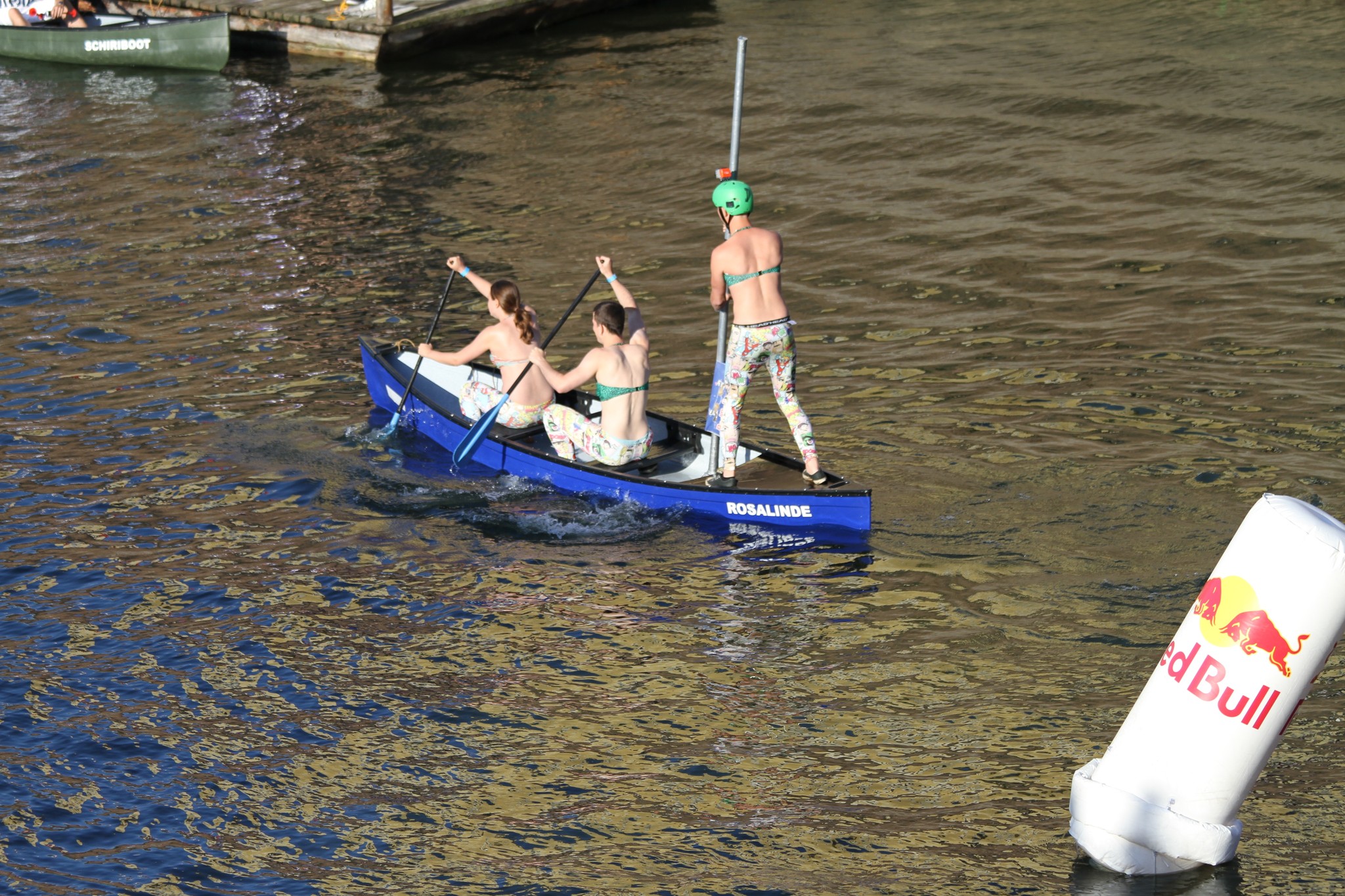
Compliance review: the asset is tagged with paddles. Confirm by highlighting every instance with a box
[380,260,456,436]
[453,259,604,469]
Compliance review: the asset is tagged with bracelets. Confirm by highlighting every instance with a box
[606,274,617,283]
[460,267,470,277]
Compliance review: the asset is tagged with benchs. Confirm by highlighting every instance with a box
[491,394,704,474]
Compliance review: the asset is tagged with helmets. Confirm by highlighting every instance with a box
[712,180,754,216]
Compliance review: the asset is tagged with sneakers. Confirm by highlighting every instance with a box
[802,468,827,485]
[639,463,658,473]
[705,473,738,489]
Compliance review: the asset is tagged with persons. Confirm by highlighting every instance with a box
[418,256,556,429]
[529,256,653,466]
[0,0,87,28]
[705,181,826,490]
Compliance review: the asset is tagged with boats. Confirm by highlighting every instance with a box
[1,0,231,74]
[357,334,872,531]
